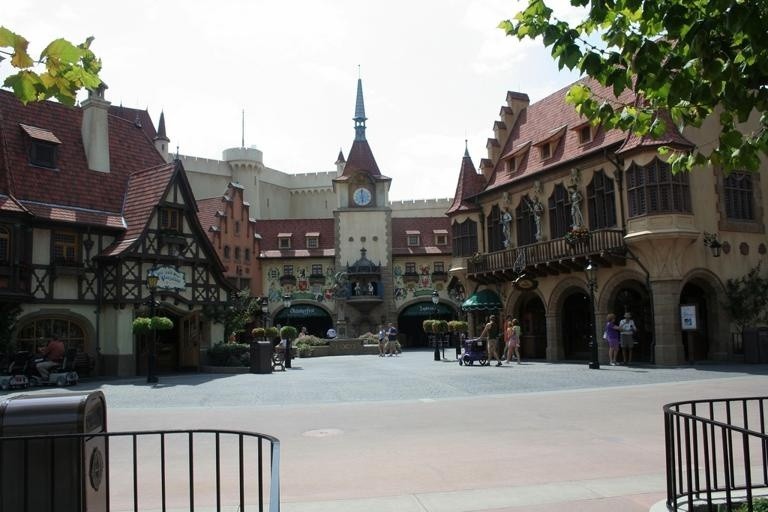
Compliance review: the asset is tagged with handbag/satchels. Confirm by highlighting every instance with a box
[603,331,612,340]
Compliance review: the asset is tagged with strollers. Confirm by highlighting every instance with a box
[458,338,488,366]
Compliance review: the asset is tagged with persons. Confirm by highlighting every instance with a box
[619,312,637,364]
[377,323,388,358]
[503,318,522,365]
[500,315,516,361]
[384,322,399,357]
[530,194,544,238]
[228,330,237,344]
[34,332,64,382]
[566,183,586,230]
[497,205,513,249]
[506,320,521,348]
[474,314,503,368]
[605,314,629,366]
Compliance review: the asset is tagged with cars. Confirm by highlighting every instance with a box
[0,368,28,390]
[29,359,79,385]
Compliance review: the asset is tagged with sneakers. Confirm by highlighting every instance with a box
[485,362,490,366]
[610,361,632,366]
[496,361,502,367]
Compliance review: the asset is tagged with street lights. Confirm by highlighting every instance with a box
[147,276,158,382]
[283,296,291,368]
[583,261,599,370]
[431,290,440,360]
[262,303,267,340]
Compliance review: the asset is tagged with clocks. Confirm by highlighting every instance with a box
[353,188,371,206]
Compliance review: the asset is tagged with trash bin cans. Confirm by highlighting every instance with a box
[743,327,768,364]
[250,341,272,374]
[0,390,109,512]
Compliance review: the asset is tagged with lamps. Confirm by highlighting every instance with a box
[705,231,722,256]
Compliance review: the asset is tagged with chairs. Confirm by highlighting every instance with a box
[51,348,77,373]
[0,351,28,376]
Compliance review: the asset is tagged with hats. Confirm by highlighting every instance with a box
[489,315,496,320]
[624,313,632,317]
